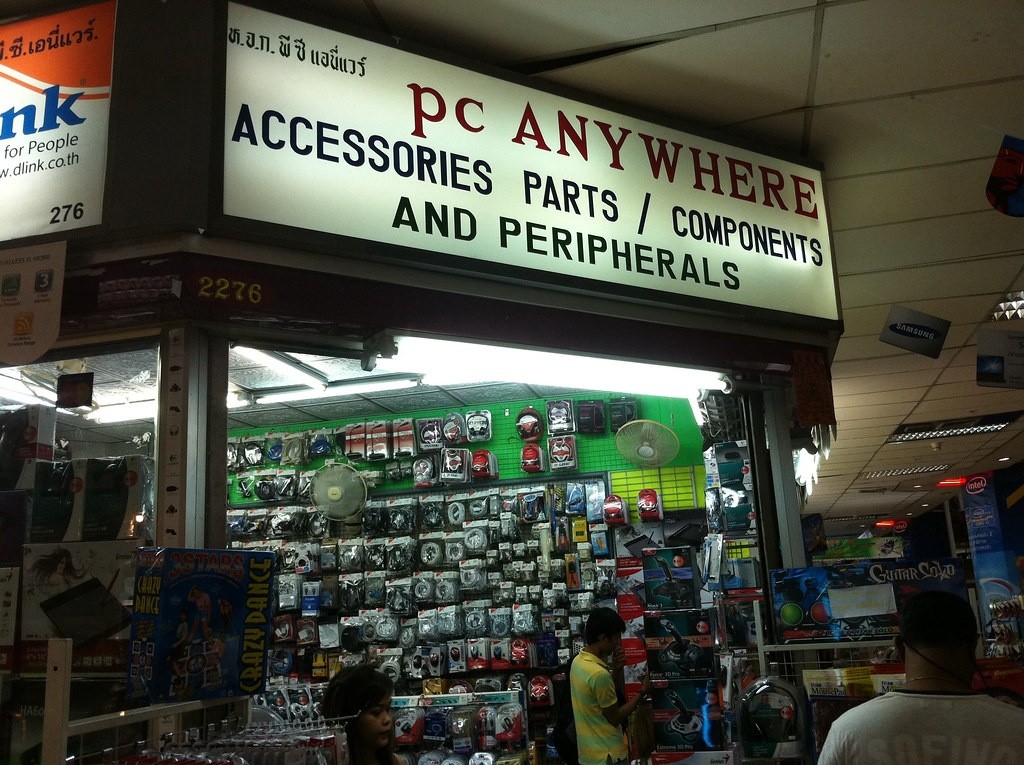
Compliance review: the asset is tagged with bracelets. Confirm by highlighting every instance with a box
[638,688,646,699]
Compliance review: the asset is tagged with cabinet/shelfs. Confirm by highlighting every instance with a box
[703,392,784,765]
[753,589,983,765]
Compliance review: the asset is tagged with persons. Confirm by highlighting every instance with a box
[320,664,408,765]
[569,607,650,765]
[817,590,1024,765]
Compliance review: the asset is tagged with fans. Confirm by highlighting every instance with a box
[614,420,680,468]
[21,358,98,417]
[309,463,368,535]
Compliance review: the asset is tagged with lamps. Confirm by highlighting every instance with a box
[229,342,328,392]
[247,373,424,408]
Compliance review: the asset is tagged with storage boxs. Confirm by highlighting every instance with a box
[614,522,665,703]
[649,666,729,752]
[652,741,742,765]
[769,557,969,646]
[0,406,158,765]
[703,440,764,646]
[588,524,608,555]
[642,544,702,609]
[643,606,722,676]
[566,480,605,523]
[124,547,275,707]
[345,418,419,462]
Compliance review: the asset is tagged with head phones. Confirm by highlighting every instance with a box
[740,680,799,743]
[413,414,490,483]
[516,400,573,471]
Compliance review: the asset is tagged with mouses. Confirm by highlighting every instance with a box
[413,646,501,669]
